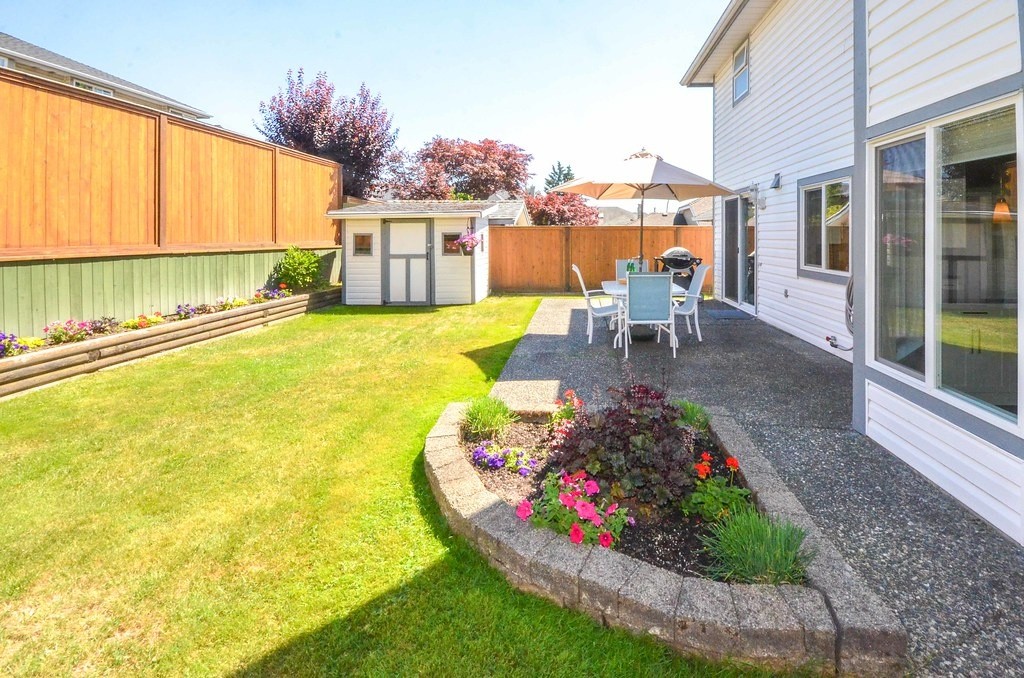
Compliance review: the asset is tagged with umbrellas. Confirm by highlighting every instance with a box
[546,148,739,273]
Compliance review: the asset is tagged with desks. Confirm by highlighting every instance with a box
[601,280,686,349]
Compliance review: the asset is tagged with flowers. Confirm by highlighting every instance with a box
[454,233,481,252]
[882,235,916,248]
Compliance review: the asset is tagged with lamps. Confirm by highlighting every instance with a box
[991,166,1012,222]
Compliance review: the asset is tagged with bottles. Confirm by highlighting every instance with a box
[626,259,636,279]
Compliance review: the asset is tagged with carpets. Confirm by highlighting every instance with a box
[705,310,752,319]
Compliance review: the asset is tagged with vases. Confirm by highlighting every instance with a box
[886,244,907,268]
[461,246,473,255]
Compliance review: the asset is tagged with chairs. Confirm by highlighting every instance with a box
[624,271,676,359]
[616,259,648,305]
[571,263,633,345]
[658,264,710,344]
